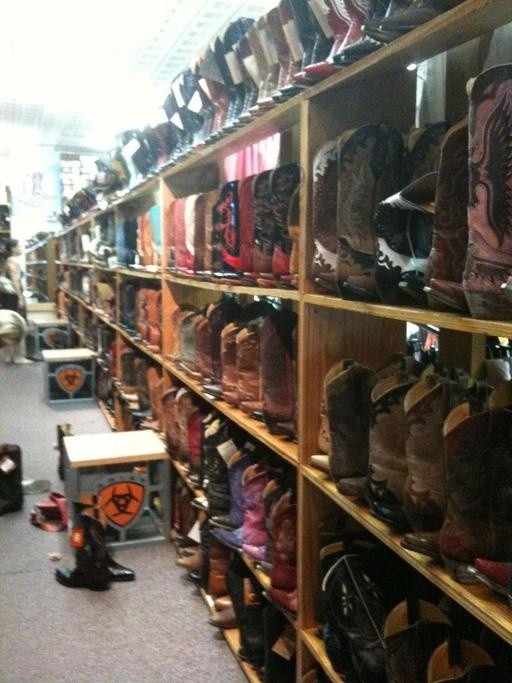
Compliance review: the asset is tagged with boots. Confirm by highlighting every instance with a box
[104,553,135,583]
[0,443,23,515]
[28,123,162,435]
[51,422,72,480]
[55,515,113,591]
[171,0,512,682]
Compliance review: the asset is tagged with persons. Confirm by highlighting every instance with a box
[0,238,41,362]
[0,307,35,362]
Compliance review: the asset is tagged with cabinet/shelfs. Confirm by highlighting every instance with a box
[25,0,512,683]
